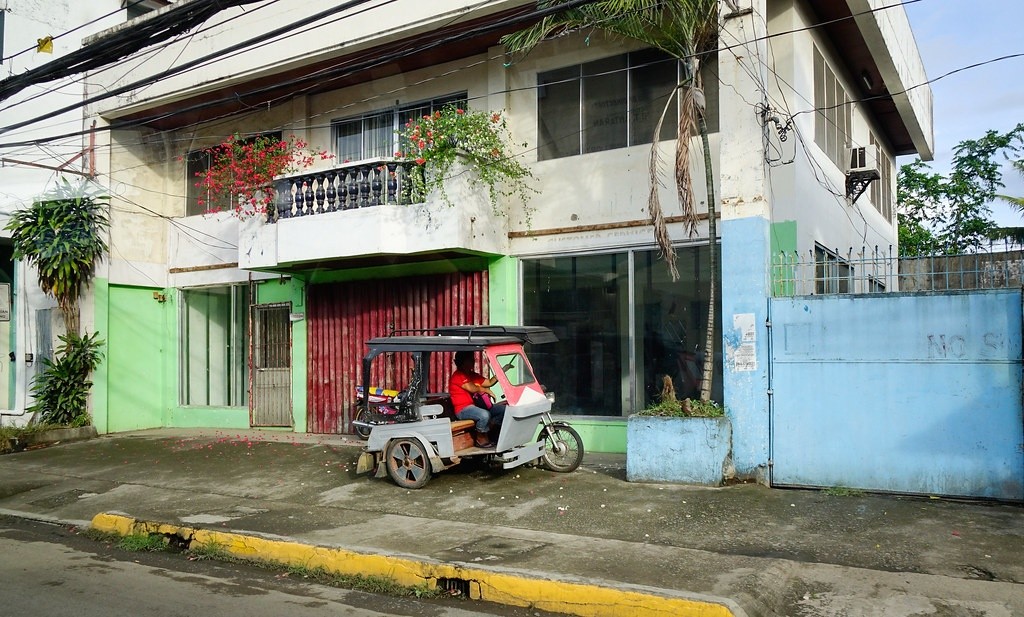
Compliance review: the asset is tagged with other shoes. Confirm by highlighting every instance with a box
[475,433,494,448]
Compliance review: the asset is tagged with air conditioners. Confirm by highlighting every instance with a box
[851,145,881,177]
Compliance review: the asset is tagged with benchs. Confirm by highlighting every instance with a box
[420,396,475,432]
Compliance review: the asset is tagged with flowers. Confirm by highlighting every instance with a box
[177,132,349,221]
[377,99,543,243]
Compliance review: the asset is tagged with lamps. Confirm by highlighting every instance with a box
[862,70,873,90]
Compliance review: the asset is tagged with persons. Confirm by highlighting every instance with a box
[448,351,515,448]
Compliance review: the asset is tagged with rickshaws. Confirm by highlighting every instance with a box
[352,326,584,489]
